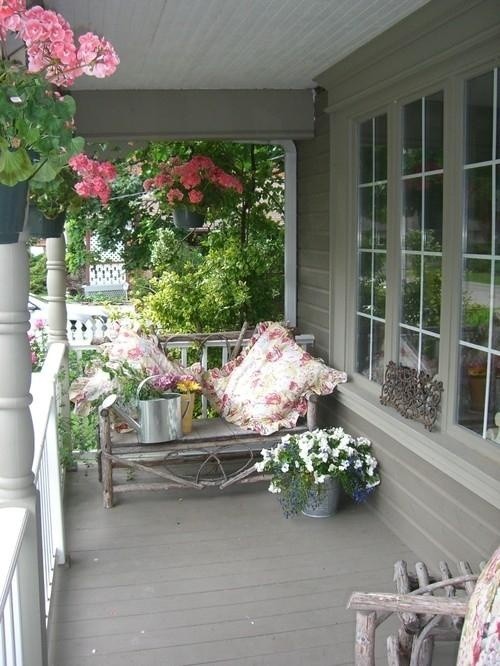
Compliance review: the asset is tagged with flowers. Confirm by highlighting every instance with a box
[0,0,120,206]
[254,425,380,520]
[172,376,201,393]
[143,154,243,215]
[467,365,488,377]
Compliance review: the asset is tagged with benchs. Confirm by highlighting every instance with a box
[97,321,318,509]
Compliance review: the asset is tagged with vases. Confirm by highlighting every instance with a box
[467,376,496,415]
[299,478,341,518]
[177,393,195,434]
[0,149,65,244]
[173,207,205,228]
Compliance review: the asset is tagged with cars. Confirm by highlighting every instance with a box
[24,291,110,340]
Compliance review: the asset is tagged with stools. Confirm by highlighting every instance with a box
[386,559,489,666]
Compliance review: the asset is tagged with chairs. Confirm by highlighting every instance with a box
[346,547,500,666]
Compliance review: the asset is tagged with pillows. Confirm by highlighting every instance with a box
[69,322,202,417]
[361,331,432,386]
[193,321,347,436]
